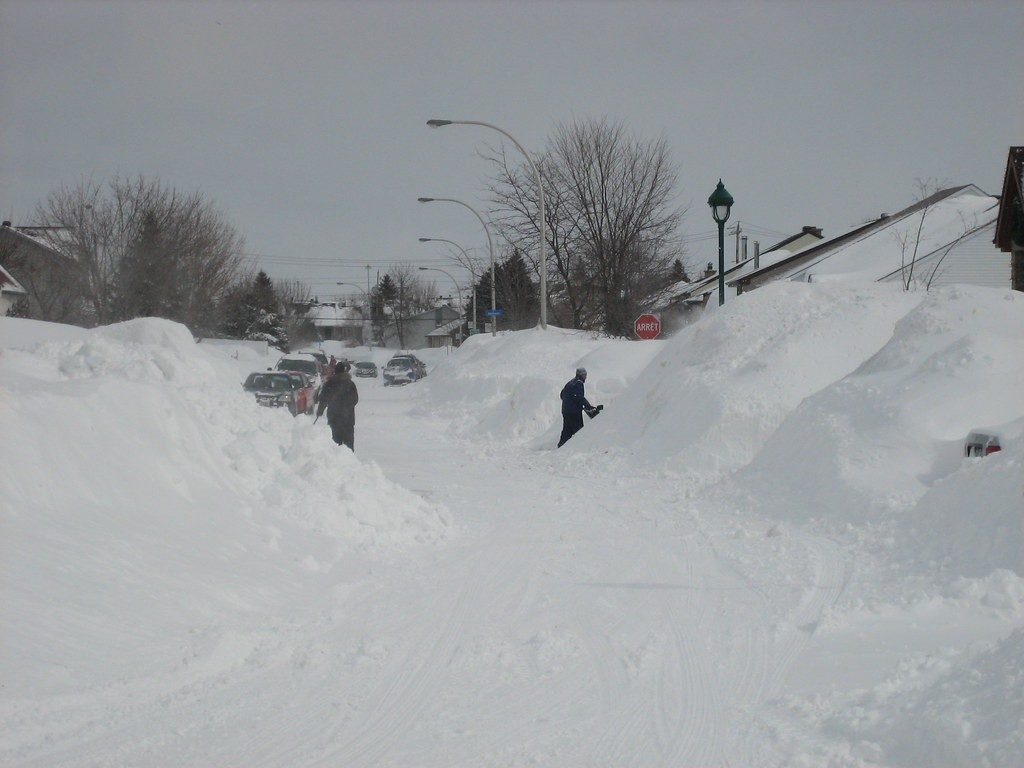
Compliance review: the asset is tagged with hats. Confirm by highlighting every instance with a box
[576,368,587,376]
[335,362,344,373]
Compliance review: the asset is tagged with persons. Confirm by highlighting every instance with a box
[556,369,606,448]
[329,347,352,376]
[317,362,359,451]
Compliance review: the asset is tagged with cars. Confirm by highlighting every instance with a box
[381,354,428,386]
[287,370,318,415]
[354,361,379,378]
[267,350,329,401]
[240,370,296,419]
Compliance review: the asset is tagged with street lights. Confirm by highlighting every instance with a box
[418,237,477,334]
[427,116,547,329]
[418,197,498,337]
[706,179,734,308]
[419,267,463,348]
[336,282,372,322]
